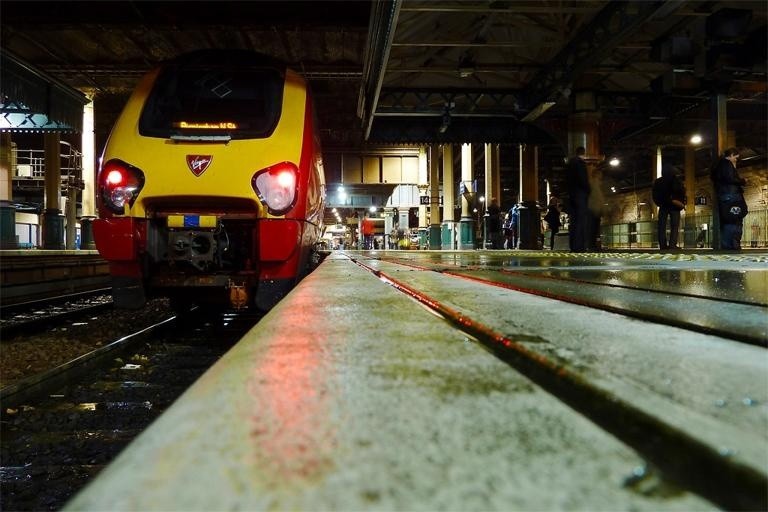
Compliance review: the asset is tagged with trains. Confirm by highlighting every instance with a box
[88,59,327,316]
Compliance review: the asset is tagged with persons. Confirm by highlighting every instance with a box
[362,214,374,248]
[489,147,606,253]
[652,165,687,250]
[711,148,749,251]
